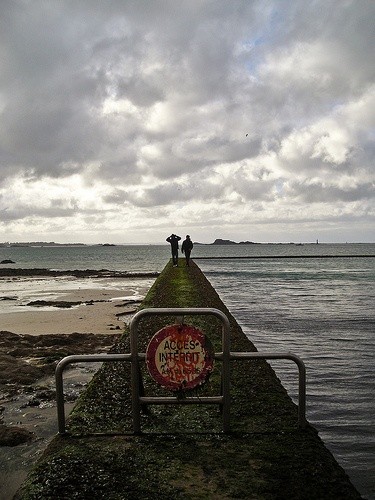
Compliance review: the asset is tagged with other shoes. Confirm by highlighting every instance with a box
[186,264,190,267]
[173,264,178,267]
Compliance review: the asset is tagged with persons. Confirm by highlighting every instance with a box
[181,234,194,267]
[166,234,181,268]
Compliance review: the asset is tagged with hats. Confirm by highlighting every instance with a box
[170,234,176,236]
